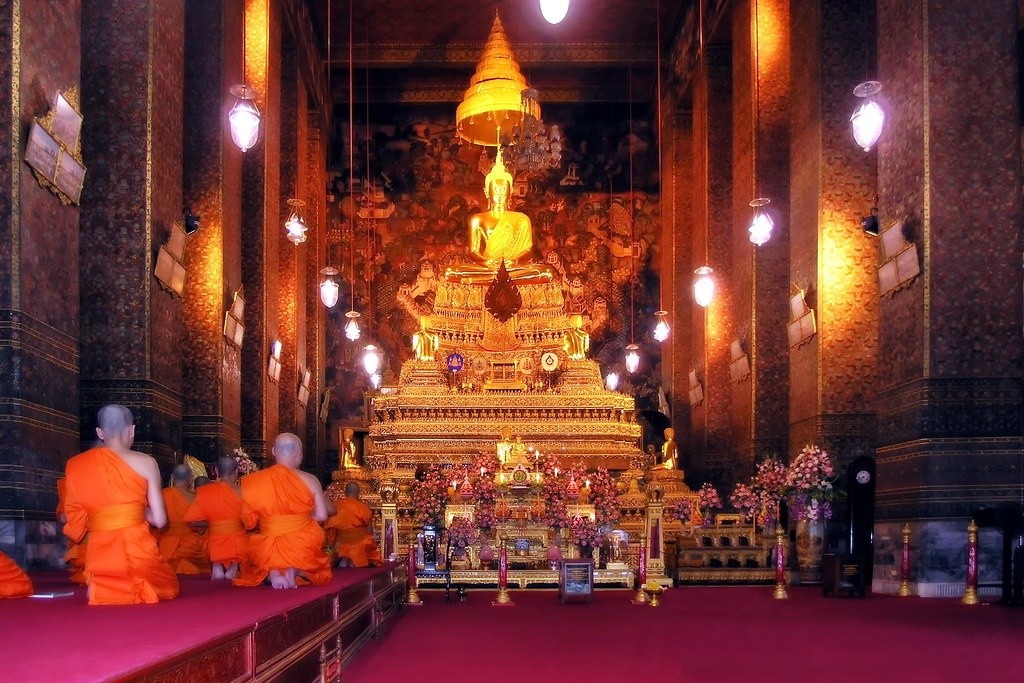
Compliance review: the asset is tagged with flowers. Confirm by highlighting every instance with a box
[410,453,834,548]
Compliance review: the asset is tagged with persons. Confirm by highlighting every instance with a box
[652,428,680,471]
[413,315,439,362]
[337,429,363,469]
[1,405,383,606]
[561,314,590,359]
[440,161,553,285]
[509,435,525,454]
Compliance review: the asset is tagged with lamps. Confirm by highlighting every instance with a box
[183,206,200,234]
[654,0,669,343]
[500,40,562,174]
[369,372,382,391]
[861,206,877,237]
[229,0,261,152]
[319,1,340,308]
[286,176,307,245]
[626,63,640,374]
[606,179,619,392]
[344,1,361,344]
[362,55,379,374]
[693,261,718,309]
[850,44,887,152]
[748,0,774,248]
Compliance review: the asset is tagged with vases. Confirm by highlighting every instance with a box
[794,517,826,584]
[423,524,439,572]
[679,519,687,537]
[480,527,494,560]
[548,528,561,561]
